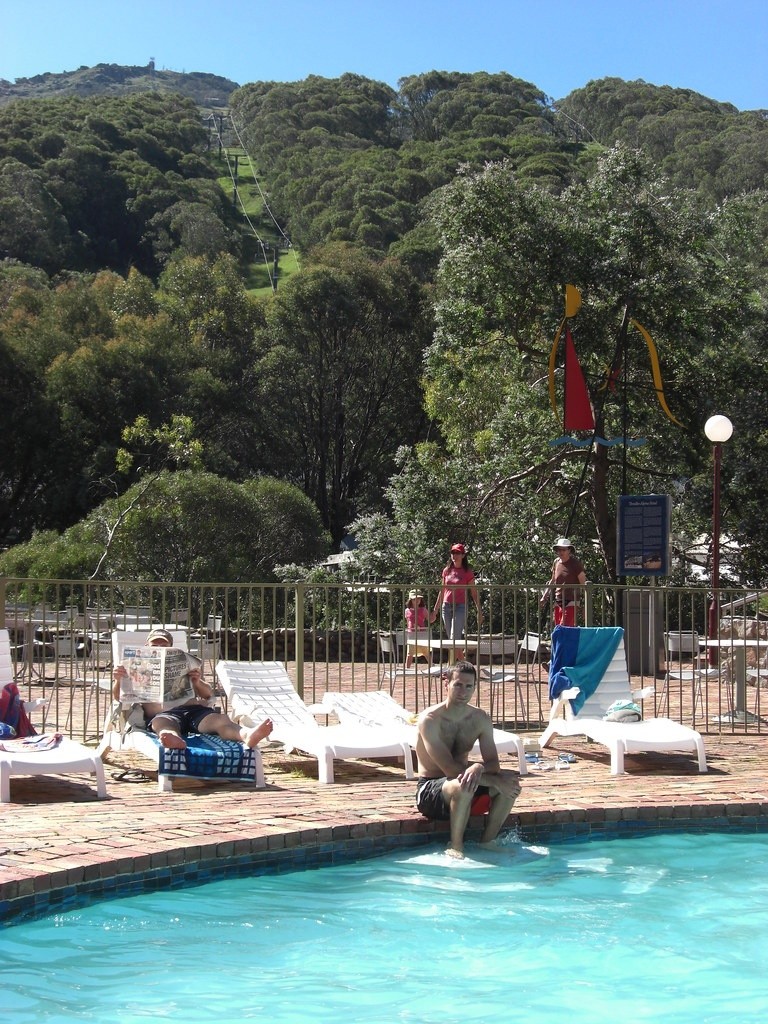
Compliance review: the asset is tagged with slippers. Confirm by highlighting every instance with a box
[532,761,554,770]
[111,768,153,783]
[555,758,570,769]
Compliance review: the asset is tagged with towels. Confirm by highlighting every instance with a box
[549,625,625,717]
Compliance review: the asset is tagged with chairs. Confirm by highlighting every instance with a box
[377,626,527,731]
[0,629,107,802]
[215,659,417,783]
[326,689,527,779]
[538,626,768,777]
[482,631,544,731]
[54,605,267,791]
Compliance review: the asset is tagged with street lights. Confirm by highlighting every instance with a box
[703,414,733,679]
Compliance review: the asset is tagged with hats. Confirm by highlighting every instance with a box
[450,544,465,554]
[408,588,423,600]
[146,628,173,644]
[552,537,576,554]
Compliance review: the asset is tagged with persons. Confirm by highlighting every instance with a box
[405,544,482,679]
[415,660,524,850]
[114,629,273,749]
[540,538,585,673]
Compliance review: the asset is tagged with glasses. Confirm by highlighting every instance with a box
[450,551,461,555]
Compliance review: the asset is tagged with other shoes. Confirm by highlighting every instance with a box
[525,752,539,763]
[542,661,550,673]
[559,752,577,762]
[439,671,448,679]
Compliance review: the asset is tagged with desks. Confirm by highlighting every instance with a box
[700,639,768,724]
[406,640,495,666]
